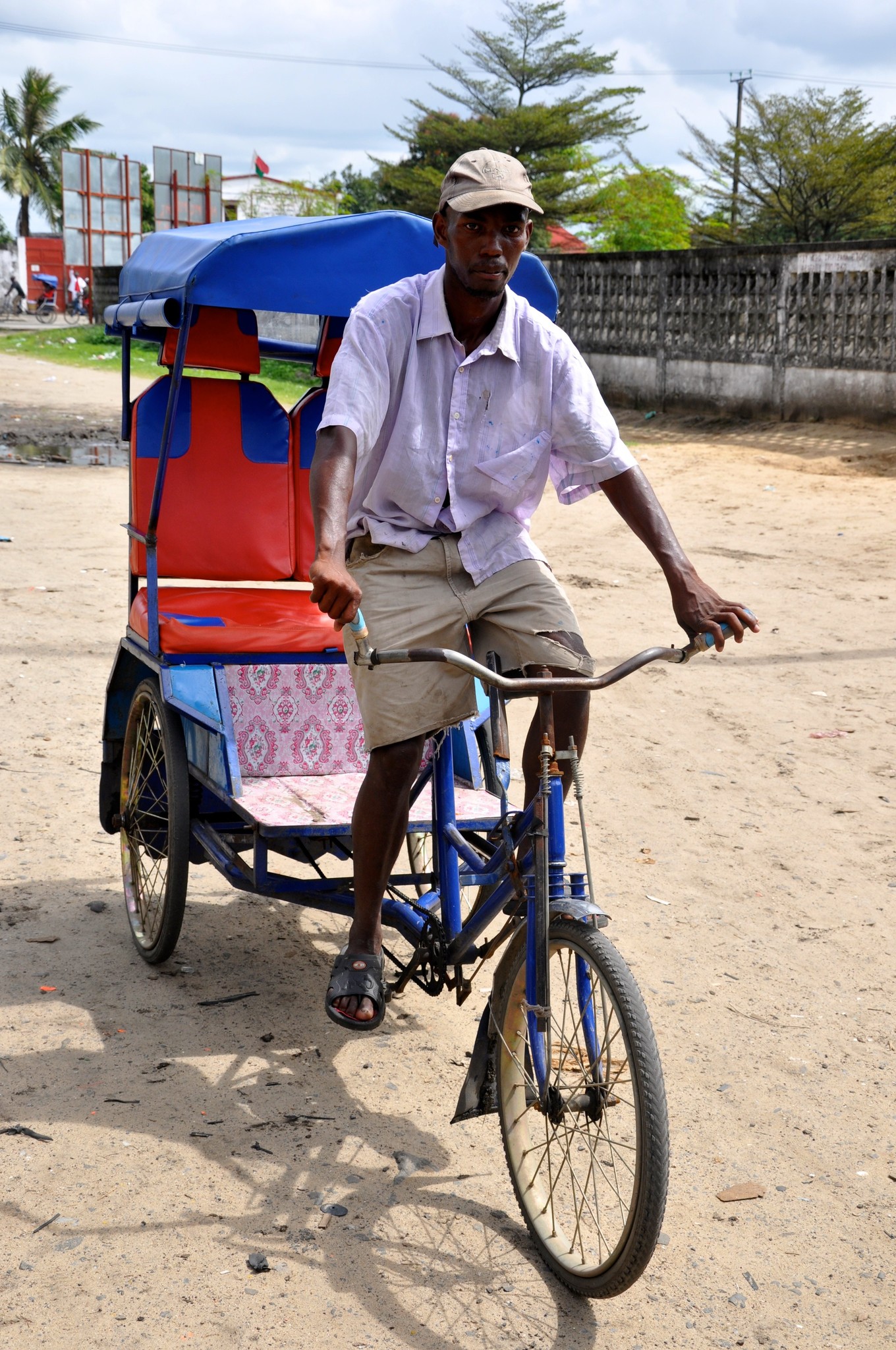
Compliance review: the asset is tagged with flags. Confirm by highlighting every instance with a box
[252,150,269,178]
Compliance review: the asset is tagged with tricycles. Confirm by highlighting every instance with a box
[64,277,89,325]
[100,206,749,1299]
[0,273,60,325]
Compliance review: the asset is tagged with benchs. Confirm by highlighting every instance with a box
[128,297,346,665]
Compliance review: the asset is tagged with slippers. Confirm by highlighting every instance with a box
[503,893,609,927]
[324,940,386,1031]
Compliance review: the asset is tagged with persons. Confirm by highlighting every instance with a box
[67,266,88,314]
[308,147,761,1031]
[5,277,25,316]
[35,281,54,311]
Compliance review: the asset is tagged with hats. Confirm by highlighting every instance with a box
[439,148,543,217]
